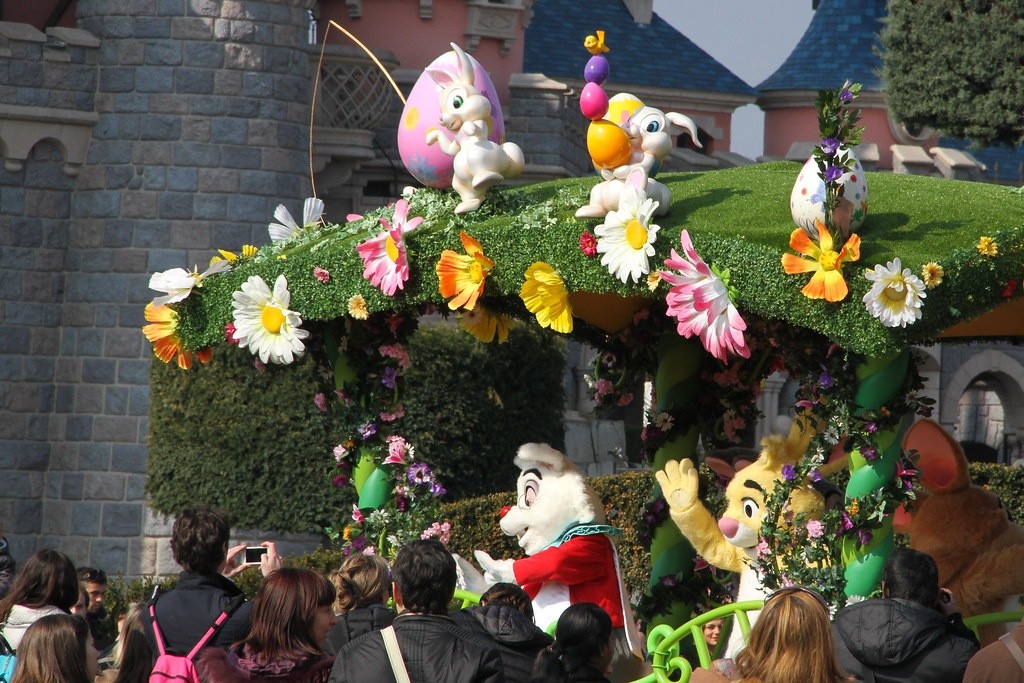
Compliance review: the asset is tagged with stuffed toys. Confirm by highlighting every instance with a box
[450,442,644,683]
[893,418,1024,649]
[655,397,843,659]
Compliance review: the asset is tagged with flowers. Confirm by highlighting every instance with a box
[579,185,663,293]
[354,198,424,296]
[583,309,938,619]
[921,260,944,291]
[314,266,329,284]
[346,294,369,321]
[659,227,751,365]
[435,231,573,344]
[268,197,324,246]
[975,235,999,259]
[779,219,861,303]
[811,80,865,245]
[141,243,309,375]
[313,307,452,570]
[863,257,927,328]
[1002,279,1016,298]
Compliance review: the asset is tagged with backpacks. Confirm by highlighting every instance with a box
[148,584,245,683]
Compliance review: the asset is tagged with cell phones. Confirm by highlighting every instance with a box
[244,547,269,565]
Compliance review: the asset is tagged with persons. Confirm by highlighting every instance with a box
[0,534,107,683]
[198,567,338,683]
[448,582,554,683]
[139,508,282,683]
[322,553,397,657]
[688,587,863,683]
[834,548,980,683]
[963,616,1024,683]
[691,618,723,666]
[328,539,504,683]
[527,602,615,683]
[95,604,153,683]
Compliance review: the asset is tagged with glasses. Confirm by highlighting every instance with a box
[764,586,829,620]
[83,569,105,580]
